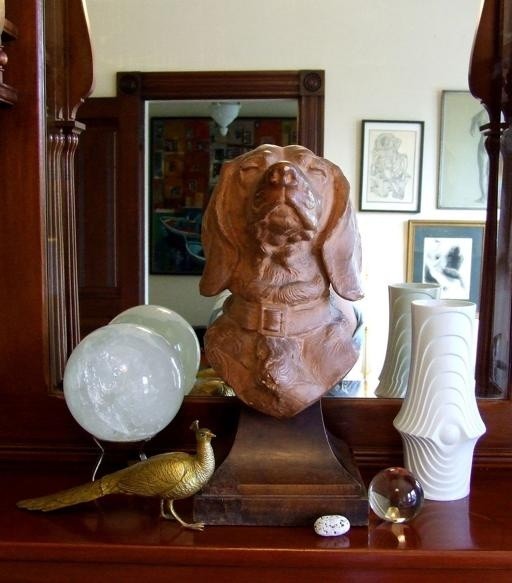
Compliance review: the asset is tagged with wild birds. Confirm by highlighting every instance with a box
[14,427,218,533]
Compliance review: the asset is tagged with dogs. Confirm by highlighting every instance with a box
[197,142,365,422]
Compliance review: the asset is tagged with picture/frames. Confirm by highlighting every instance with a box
[404,220,486,313]
[146,116,297,276]
[435,89,490,210]
[359,120,424,213]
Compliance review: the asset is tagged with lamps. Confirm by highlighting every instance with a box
[207,99,241,139]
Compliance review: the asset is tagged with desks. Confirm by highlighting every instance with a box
[1,459,512,582]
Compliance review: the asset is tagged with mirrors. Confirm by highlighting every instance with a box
[43,0,512,477]
